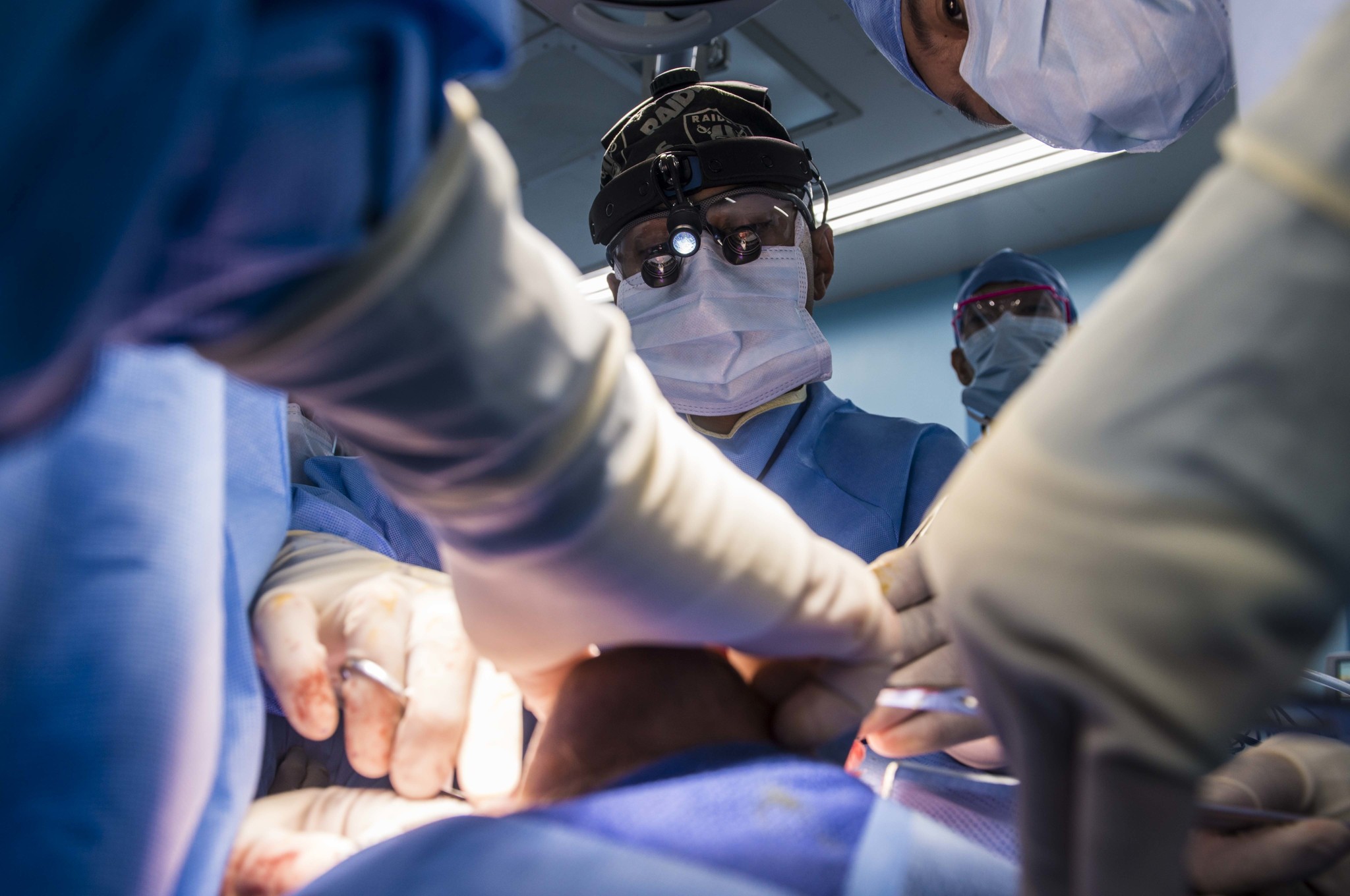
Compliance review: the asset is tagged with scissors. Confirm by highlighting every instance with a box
[338,658,466,796]
[868,683,992,717]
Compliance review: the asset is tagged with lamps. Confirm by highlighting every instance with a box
[654,151,701,254]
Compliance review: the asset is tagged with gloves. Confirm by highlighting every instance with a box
[193,0,1349,896]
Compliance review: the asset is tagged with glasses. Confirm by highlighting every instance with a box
[951,285,1071,344]
[605,187,816,288]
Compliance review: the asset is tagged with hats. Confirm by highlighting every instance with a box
[589,67,829,247]
[950,248,1079,347]
[843,0,953,108]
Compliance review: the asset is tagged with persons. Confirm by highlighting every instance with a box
[0,0,1350,896]
[950,247,1079,436]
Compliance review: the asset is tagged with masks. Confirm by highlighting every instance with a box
[618,195,833,416]
[959,0,1236,154]
[962,311,1070,425]
